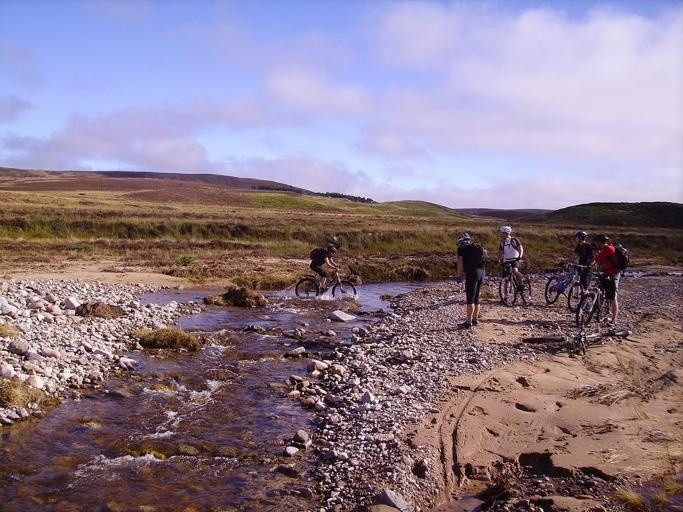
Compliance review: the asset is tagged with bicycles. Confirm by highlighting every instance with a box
[498,257,531,306]
[575,272,611,327]
[544,260,590,314]
[296,263,359,304]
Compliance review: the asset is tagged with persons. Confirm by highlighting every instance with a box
[589,233,622,325]
[455,233,485,329]
[309,246,341,291]
[497,225,524,303]
[566,232,593,291]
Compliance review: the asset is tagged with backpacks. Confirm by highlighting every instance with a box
[606,243,630,269]
[310,249,325,259]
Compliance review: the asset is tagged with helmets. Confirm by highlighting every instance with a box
[327,246,337,252]
[576,232,587,239]
[456,233,470,245]
[499,226,512,234]
[593,234,609,242]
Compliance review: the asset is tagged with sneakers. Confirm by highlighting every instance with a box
[472,319,479,325]
[458,322,472,327]
[606,321,616,326]
